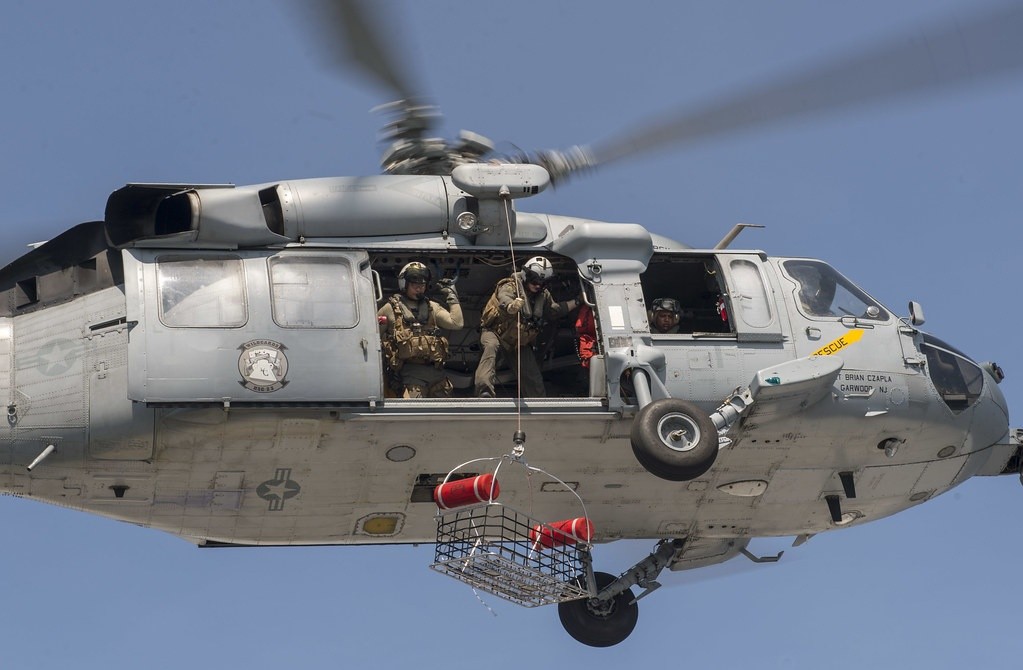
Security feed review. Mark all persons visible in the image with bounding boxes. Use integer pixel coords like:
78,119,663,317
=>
378,262,464,398
574,304,599,397
798,278,836,317
648,297,681,333
475,256,591,398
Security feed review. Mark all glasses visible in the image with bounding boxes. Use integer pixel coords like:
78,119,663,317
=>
527,278,547,286
408,280,427,289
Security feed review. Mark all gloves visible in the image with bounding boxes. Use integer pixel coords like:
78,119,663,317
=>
575,288,592,306
436,278,460,304
510,298,525,311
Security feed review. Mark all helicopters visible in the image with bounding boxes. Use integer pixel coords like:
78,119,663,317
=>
0,0,1023,647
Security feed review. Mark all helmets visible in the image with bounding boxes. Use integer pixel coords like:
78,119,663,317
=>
398,262,432,291
521,256,554,287
648,298,683,326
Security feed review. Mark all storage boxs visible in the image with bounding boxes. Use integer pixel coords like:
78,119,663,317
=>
426,502,598,610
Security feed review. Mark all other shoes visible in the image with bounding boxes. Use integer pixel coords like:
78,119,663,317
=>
479,386,495,398
